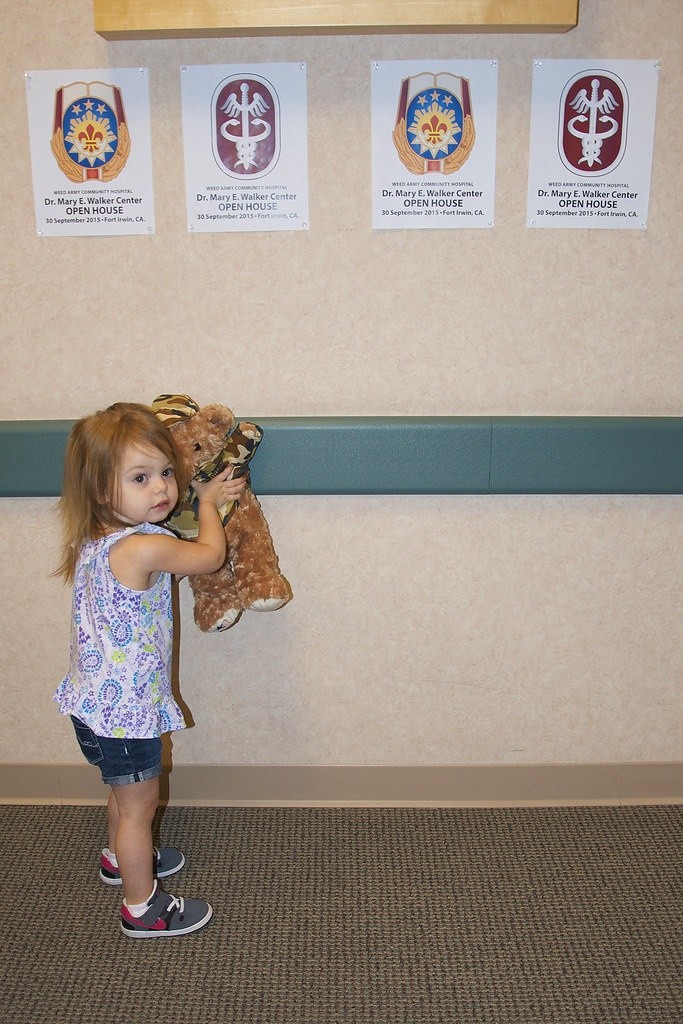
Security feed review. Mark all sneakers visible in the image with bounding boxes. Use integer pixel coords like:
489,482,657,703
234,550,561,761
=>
120,878,213,938
99,845,186,885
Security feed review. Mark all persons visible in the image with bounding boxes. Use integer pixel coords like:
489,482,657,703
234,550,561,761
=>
50,402,250,940
